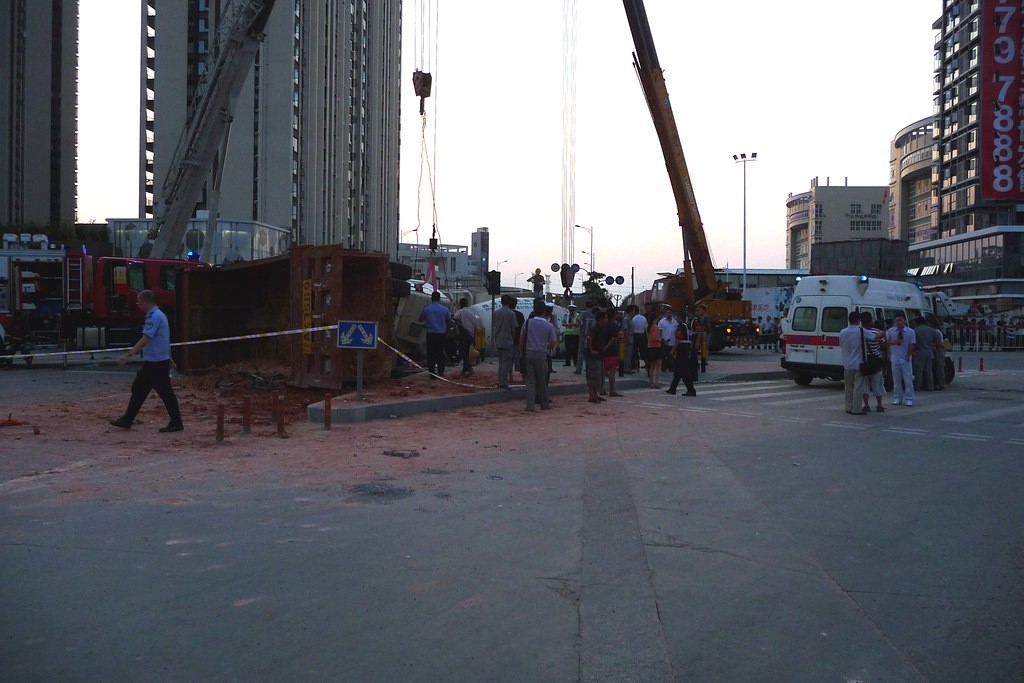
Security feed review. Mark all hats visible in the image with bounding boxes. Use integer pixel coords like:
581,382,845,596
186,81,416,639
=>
684,305,697,311
568,304,577,309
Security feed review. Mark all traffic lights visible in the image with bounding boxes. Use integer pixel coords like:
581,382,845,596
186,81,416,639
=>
483,270,500,296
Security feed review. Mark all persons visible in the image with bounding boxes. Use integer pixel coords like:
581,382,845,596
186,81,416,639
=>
561,305,580,367
943,313,1024,352
109,290,184,433
575,297,711,403
838,311,949,415
492,295,557,411
752,307,789,353
452,298,476,374
527,268,545,298
419,292,452,380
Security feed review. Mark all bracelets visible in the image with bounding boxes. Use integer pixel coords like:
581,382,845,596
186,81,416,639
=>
128,352,132,357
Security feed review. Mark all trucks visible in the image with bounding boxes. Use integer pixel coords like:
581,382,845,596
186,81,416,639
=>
175,243,411,387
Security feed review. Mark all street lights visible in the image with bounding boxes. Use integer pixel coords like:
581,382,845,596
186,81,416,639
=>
497,260,507,271
583,251,594,273
515,273,523,288
733,153,757,300
575,225,592,280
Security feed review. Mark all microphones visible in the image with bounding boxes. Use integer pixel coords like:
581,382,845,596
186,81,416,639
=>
897,332,903,345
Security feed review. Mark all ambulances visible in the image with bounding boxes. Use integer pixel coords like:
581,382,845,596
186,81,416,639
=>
781,275,943,386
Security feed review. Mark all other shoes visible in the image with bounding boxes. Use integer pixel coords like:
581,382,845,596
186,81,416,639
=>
893,398,902,404
160,420,183,432
430,368,557,411
863,406,870,411
109,419,131,429
563,362,624,403
878,405,884,411
905,400,912,405
650,364,707,396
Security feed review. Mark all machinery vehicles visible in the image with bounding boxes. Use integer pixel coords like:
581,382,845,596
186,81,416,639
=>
394,279,568,364
0,0,437,363
622,0,760,351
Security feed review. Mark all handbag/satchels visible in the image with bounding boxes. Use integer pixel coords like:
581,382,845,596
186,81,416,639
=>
860,362,872,374
469,342,480,360
520,356,526,373
